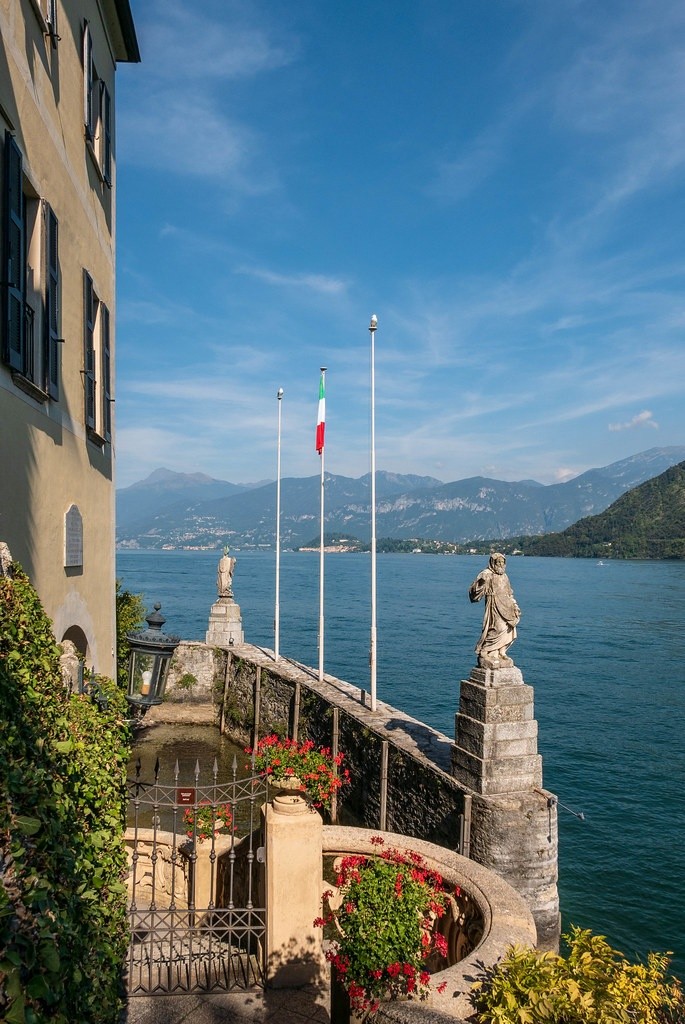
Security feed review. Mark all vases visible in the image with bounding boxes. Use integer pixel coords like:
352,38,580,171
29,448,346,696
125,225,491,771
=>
268,772,304,789
198,818,224,830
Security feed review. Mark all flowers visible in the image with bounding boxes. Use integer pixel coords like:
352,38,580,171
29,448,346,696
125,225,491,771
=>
182,800,238,844
312,837,461,1019
245,734,351,813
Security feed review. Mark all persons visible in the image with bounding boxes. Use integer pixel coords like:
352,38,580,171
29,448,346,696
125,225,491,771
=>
469,553,521,659
216,546,236,604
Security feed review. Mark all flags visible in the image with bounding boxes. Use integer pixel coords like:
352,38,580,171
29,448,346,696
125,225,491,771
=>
316,374,325,455
